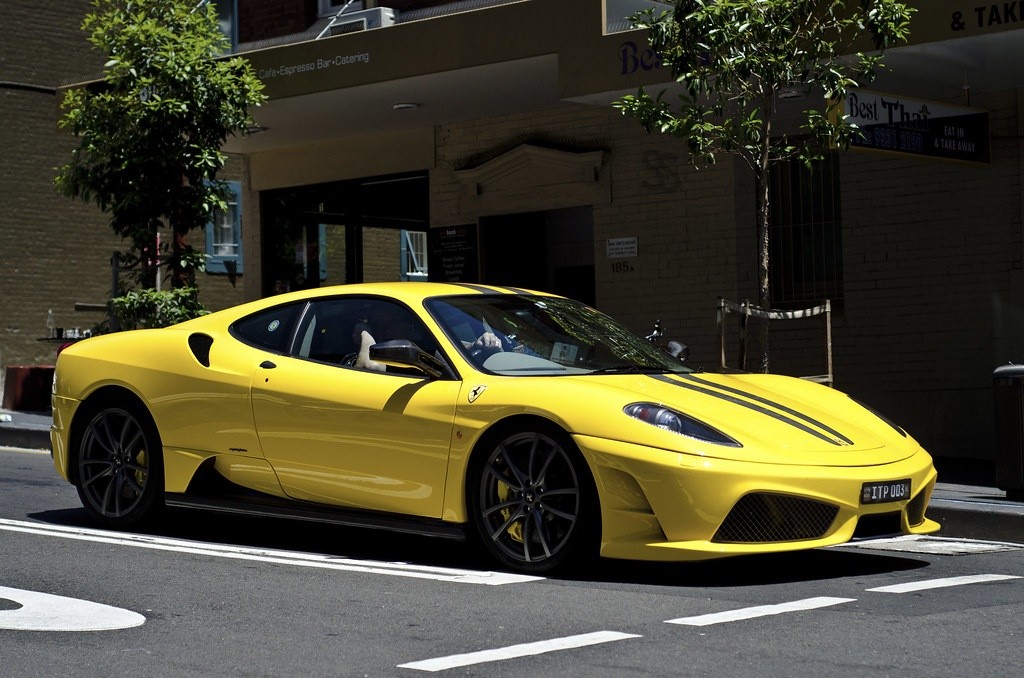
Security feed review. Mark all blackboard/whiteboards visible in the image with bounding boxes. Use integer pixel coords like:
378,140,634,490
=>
426,223,479,285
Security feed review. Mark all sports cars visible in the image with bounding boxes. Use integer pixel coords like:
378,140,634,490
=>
46,283,941,574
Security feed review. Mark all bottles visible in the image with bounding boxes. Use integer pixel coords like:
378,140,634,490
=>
45,310,54,338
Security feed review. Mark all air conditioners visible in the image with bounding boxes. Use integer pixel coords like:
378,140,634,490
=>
326,7,395,37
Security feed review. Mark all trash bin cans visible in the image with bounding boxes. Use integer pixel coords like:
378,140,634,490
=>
991,363,1024,503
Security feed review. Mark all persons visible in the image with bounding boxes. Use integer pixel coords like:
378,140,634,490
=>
352,306,503,374
